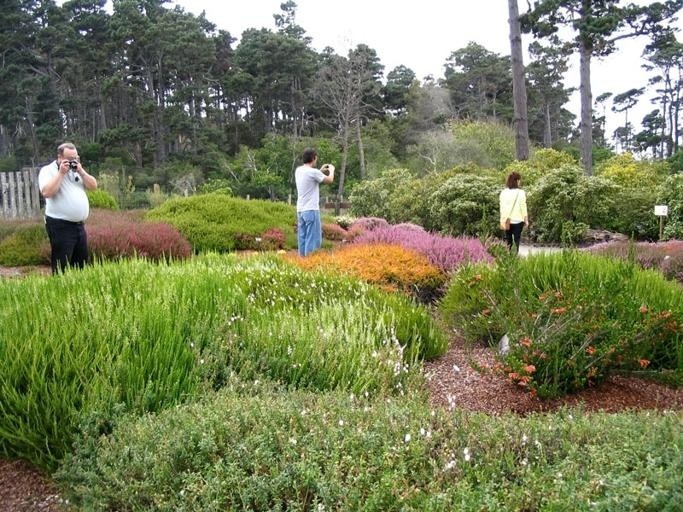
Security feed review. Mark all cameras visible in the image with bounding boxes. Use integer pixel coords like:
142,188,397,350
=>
65,161,78,169
325,166,329,168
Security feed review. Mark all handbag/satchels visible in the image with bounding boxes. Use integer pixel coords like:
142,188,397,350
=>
499,217,511,230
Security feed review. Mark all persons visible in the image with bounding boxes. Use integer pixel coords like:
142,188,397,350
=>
295,149,335,256
38,143,97,276
499,172,528,252
